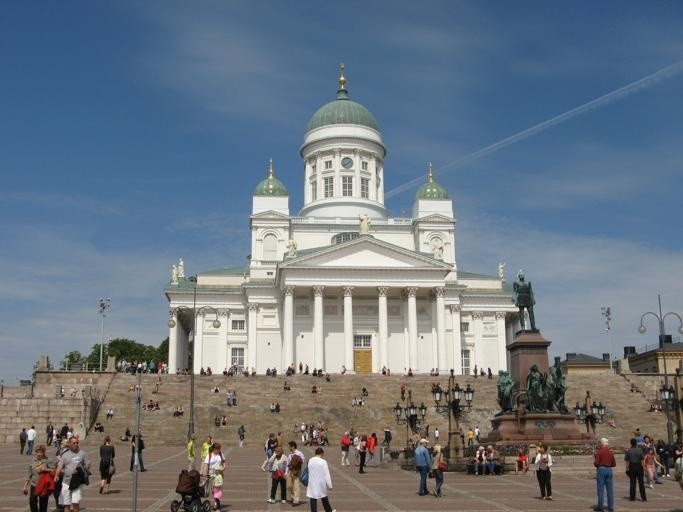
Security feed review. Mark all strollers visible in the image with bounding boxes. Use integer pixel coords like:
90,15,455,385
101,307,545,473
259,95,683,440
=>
171,468,212,512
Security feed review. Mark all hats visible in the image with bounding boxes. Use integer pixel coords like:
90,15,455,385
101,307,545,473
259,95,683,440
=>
420,438,429,443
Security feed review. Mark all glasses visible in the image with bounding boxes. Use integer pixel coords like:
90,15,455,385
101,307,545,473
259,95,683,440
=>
72,442,79,445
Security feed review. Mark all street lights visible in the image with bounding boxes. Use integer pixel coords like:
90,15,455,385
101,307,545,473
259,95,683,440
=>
164,286,220,443
600,304,616,375
573,393,606,448
98,295,110,371
390,388,426,468
434,381,475,466
648,385,676,462
130,367,142,511
633,291,683,450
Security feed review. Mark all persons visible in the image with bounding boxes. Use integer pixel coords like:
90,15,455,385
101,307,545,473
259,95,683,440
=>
473,364,492,379
186,435,226,512
260,420,337,512
352,396,365,418
609,414,617,428
172,265,177,283
496,365,567,414
380,365,441,403
270,401,281,420
61,386,79,397
432,238,444,261
238,424,246,449
499,262,506,279
340,427,392,474
592,428,683,512
105,377,187,419
177,258,184,277
117,358,191,377
458,425,554,501
513,270,539,331
359,214,371,234
124,426,147,472
411,425,448,497
286,236,297,256
212,384,238,427
264,362,368,395
20,421,115,511
199,365,257,373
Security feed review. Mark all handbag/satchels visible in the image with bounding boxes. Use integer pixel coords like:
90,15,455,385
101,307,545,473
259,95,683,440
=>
108,464,115,475
300,469,308,484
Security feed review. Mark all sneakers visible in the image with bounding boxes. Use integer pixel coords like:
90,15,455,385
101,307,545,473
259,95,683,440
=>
265,498,275,504
326,509,337,512
290,503,300,507
547,495,552,501
592,506,604,512
431,489,439,499
280,499,286,504
648,484,655,489
539,497,545,501
606,508,614,512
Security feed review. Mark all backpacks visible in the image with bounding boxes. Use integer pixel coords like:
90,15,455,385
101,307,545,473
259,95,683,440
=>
437,463,446,472
